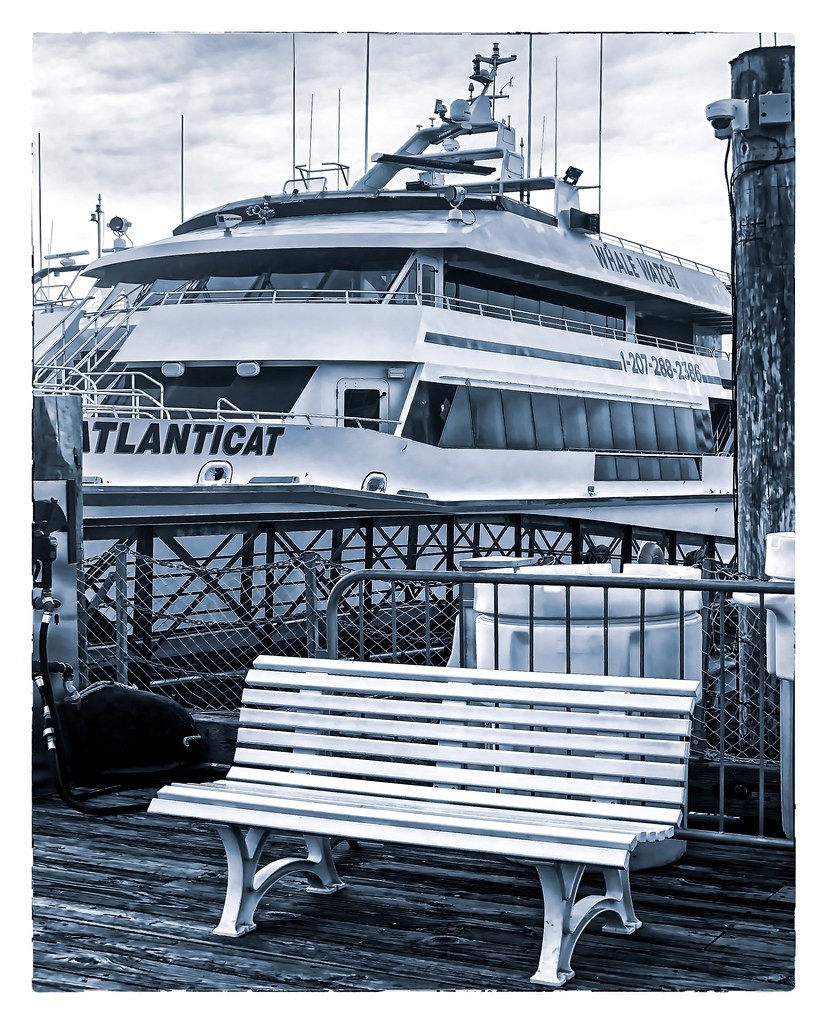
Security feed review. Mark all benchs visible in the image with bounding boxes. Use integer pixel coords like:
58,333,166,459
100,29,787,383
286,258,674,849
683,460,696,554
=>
147,651,702,986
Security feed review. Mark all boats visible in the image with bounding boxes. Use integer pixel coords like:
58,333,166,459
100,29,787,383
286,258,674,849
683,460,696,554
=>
33,29,739,652
